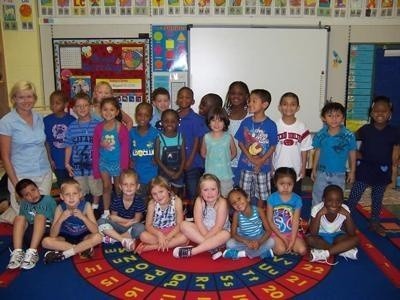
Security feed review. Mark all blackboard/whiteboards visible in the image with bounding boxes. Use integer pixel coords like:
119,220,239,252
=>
186,25,330,134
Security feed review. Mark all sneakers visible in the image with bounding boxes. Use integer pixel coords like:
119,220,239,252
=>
223,249,238,259
309,249,339,265
19,248,39,270
260,249,274,259
44,251,64,263
173,246,194,258
79,247,94,259
102,235,111,244
368,224,387,237
122,238,136,251
339,247,358,260
211,248,222,260
6,249,25,269
